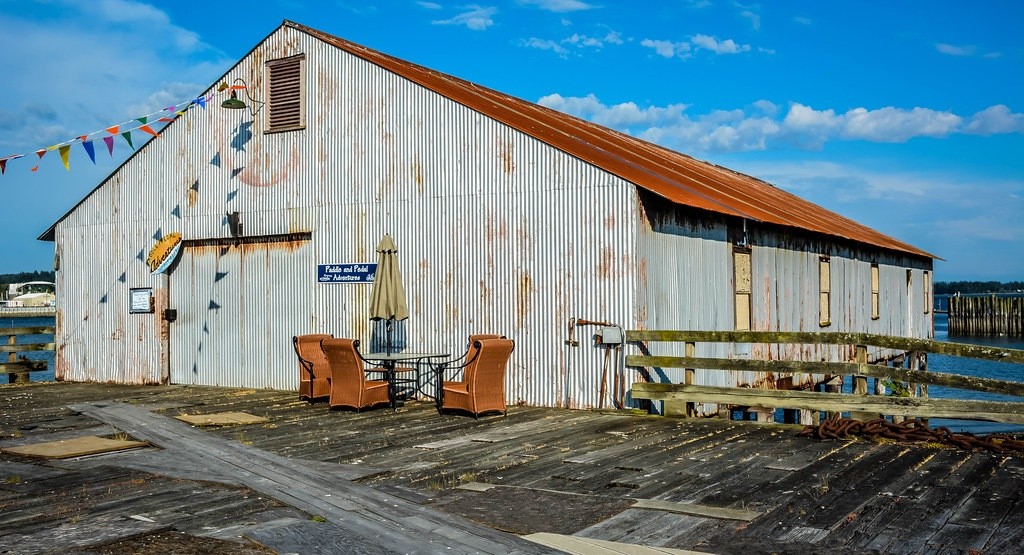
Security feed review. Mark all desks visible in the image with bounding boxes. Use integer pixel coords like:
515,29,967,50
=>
363,353,450,410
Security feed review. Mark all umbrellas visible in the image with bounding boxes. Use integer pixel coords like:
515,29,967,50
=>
370,233,409,355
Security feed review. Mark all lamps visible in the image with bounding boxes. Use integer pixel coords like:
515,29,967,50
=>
221,78,265,109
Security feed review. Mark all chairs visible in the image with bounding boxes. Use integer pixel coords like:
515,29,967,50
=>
293,334,391,413
435,334,515,421
